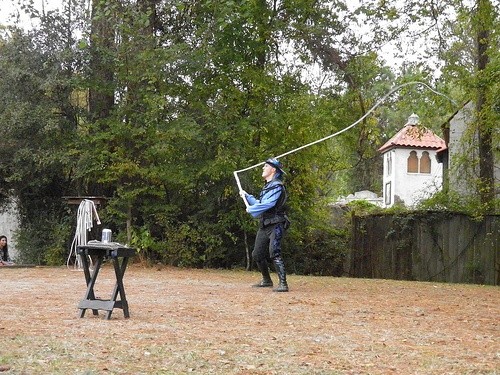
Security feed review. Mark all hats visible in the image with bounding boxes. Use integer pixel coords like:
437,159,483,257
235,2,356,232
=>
267,159,287,175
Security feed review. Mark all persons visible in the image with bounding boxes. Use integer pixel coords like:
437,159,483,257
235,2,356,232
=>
0,235,11,267
241,159,289,293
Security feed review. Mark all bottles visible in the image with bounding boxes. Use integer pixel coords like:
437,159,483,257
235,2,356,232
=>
101,228,111,244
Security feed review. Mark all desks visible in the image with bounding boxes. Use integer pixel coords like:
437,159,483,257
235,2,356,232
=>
75,247,136,317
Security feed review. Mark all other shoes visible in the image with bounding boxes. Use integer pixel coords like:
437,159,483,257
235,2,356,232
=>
254,280,273,287
272,285,288,292
